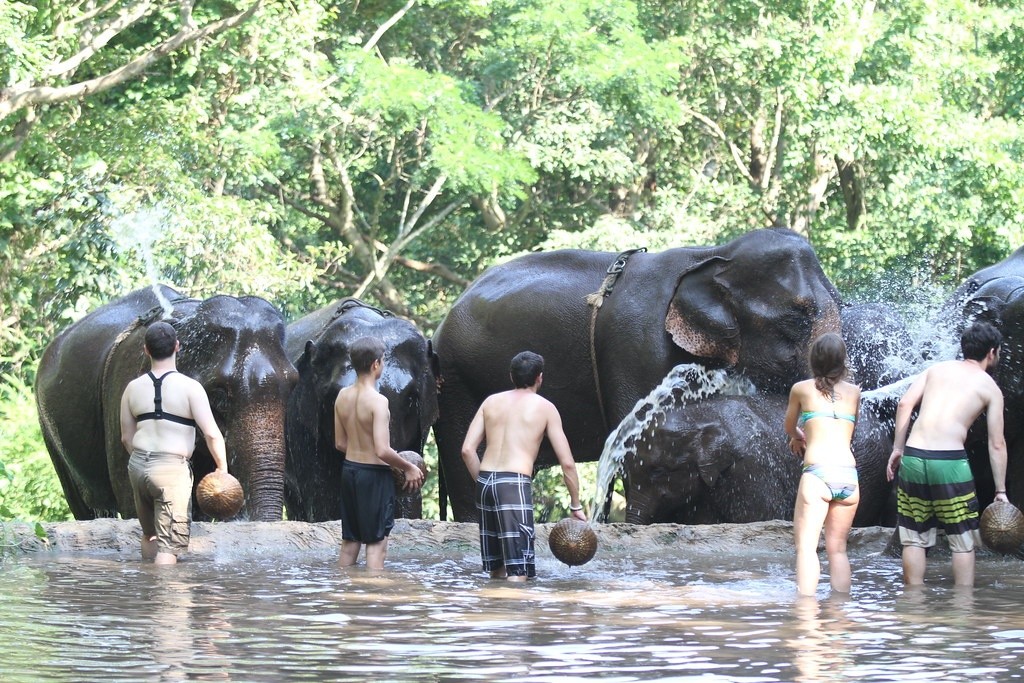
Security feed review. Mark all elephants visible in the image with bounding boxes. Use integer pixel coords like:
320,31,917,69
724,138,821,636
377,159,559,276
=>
598,244,1024,527
285,296,443,523
34,283,301,523
431,219,856,525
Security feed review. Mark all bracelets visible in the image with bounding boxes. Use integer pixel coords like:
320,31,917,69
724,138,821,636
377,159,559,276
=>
995,489,1006,494
796,434,806,442
893,449,905,453
568,504,583,511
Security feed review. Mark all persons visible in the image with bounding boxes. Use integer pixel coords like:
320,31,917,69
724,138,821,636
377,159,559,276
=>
887,322,1010,587
332,336,425,572
783,332,862,596
460,350,588,582
119,320,228,565
